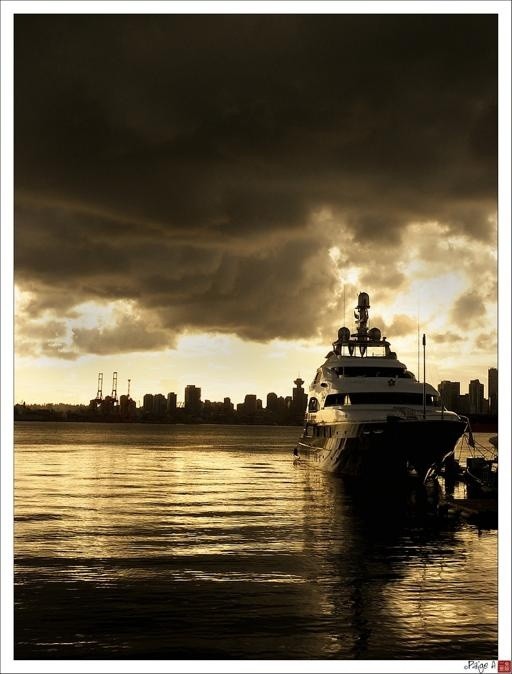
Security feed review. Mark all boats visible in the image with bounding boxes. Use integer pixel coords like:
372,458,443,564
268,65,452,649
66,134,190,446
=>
293,291,470,475
